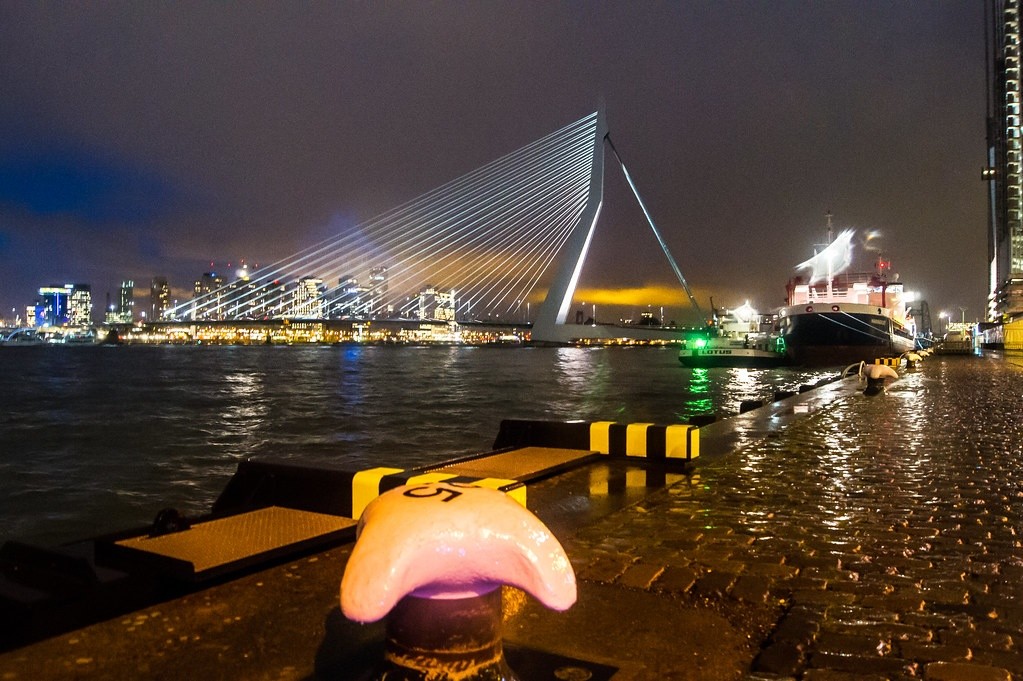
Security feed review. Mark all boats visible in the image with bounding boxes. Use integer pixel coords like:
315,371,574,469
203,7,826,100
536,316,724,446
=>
0,316,124,350
678,249,779,367
775,252,986,375
